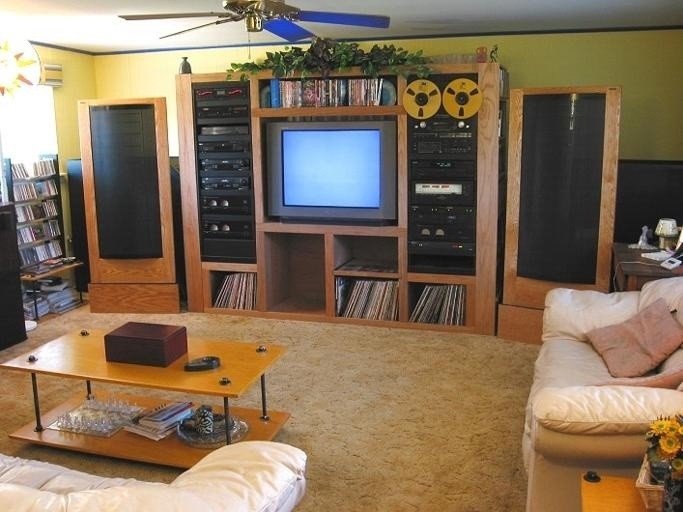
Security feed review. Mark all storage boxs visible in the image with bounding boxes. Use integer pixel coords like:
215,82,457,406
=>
103,320,188,369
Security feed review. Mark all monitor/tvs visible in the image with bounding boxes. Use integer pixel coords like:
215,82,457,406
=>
266,121,396,225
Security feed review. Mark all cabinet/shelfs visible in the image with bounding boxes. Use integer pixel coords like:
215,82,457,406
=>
173,63,499,336
2,152,66,267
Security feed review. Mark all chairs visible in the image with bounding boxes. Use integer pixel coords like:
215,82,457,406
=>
0,440,305,511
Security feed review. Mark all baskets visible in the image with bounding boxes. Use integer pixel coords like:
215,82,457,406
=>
635,450,667,511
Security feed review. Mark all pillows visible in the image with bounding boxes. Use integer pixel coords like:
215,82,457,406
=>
582,296,682,388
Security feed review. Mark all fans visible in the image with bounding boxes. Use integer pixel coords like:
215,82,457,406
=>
118,0,390,44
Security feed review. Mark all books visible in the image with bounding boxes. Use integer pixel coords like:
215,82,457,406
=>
124,399,195,443
271,78,384,108
212,273,256,311
335,276,465,325
10,158,84,319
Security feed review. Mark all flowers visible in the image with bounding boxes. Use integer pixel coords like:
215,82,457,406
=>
646,412,683,482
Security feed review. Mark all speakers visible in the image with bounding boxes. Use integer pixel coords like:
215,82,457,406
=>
77,97,177,284
502,84,622,311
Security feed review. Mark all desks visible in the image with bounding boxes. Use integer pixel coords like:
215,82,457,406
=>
20,260,86,322
611,242,682,291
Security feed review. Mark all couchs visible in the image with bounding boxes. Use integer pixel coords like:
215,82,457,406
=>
517,276,682,511
